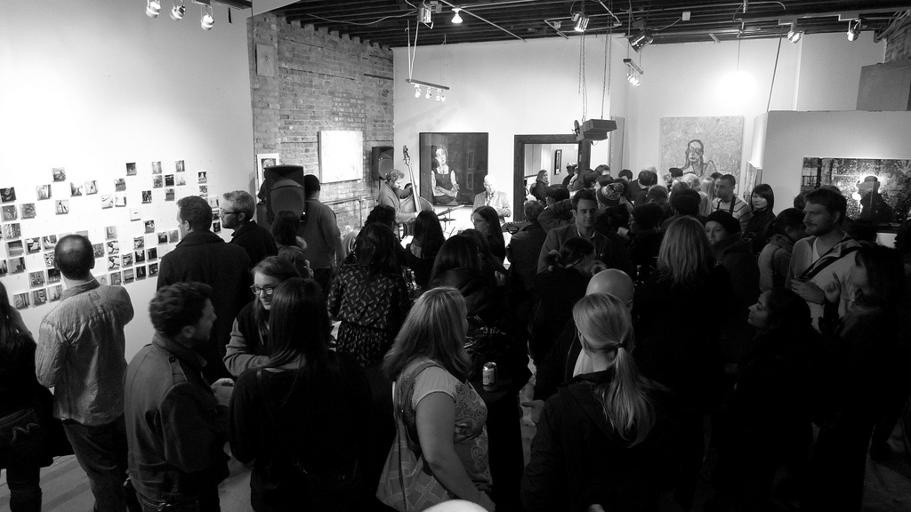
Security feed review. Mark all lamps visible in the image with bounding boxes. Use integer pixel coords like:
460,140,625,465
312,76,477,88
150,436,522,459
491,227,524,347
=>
451,6,464,25
629,31,655,54
787,23,807,46
572,10,591,35
407,79,451,103
838,11,866,43
623,58,645,88
144,0,216,32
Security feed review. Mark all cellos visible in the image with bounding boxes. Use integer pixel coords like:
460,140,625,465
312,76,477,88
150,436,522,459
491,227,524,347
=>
399,145,434,259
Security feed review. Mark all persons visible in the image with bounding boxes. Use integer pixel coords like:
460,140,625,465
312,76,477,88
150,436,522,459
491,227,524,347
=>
156,196,255,486
34,234,143,511
430,144,461,206
219,163,911,510
124,282,236,511
0,282,76,512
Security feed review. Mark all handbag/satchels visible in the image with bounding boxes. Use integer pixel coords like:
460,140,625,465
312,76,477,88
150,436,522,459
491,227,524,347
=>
375,418,455,512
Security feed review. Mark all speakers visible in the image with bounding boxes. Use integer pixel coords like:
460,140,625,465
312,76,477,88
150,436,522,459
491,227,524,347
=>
372,146,394,181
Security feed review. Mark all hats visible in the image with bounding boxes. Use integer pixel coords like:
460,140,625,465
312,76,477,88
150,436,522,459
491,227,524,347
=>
703,210,737,233
596,182,625,207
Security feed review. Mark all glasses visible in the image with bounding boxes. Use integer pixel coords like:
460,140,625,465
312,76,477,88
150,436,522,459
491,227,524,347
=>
217,208,242,214
250,282,281,296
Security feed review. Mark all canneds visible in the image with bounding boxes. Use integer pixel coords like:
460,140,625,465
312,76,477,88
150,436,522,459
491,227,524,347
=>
483,361,498,392
